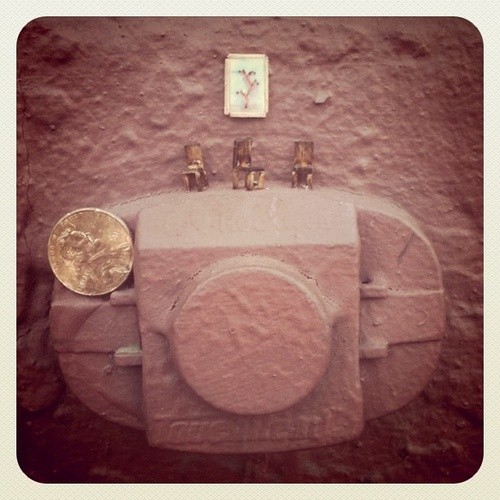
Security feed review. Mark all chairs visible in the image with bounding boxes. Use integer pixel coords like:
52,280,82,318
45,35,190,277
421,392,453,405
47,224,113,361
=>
180,142,207,191
231,138,264,190
291,141,313,189
